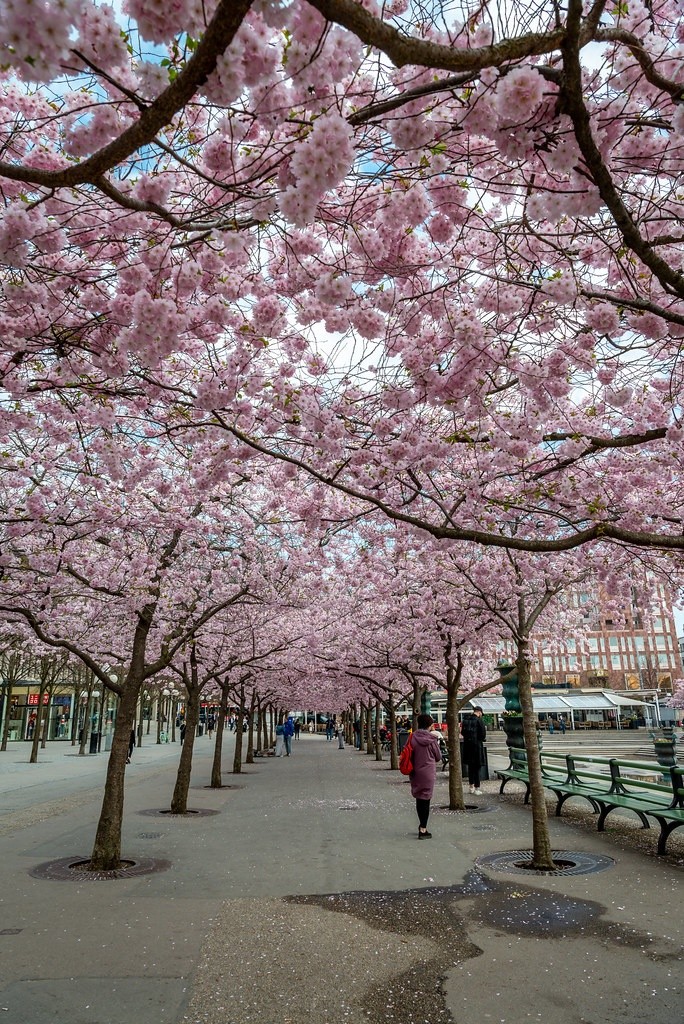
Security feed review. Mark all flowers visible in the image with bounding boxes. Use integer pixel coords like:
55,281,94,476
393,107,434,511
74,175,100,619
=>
653,738,672,744
503,710,518,717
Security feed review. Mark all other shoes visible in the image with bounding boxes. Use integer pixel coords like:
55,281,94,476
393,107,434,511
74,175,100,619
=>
419,830,432,840
469,786,482,796
342,731,345,735
126,758,131,764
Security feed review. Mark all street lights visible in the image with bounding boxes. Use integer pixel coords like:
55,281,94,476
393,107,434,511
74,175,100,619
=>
108,697,112,721
163,681,180,743
142,690,150,717
199,695,211,734
79,689,100,714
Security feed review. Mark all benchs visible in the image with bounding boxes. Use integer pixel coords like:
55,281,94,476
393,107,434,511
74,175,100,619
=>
519,777,564,804
548,785,608,814
647,808,684,856
590,794,668,830
540,751,601,814
567,753,650,829
612,759,683,807
508,746,564,800
494,770,528,794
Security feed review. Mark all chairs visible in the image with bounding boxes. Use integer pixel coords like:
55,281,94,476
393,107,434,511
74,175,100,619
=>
624,721,629,728
586,721,591,729
567,722,571,730
597,722,604,729
606,722,612,729
578,722,584,729
318,724,322,732
321,724,328,731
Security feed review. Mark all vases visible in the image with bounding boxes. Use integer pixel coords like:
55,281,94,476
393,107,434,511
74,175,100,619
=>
651,733,677,782
501,713,527,770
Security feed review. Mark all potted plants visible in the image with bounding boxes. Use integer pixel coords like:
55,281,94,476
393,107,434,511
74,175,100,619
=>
494,660,521,714
399,730,411,755
483,714,495,730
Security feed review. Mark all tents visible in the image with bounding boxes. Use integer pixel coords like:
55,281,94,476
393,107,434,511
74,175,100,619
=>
468,693,659,732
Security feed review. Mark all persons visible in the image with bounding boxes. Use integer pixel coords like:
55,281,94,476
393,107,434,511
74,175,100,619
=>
633,713,636,719
356,716,360,748
308,720,314,734
176,718,179,727
274,717,301,758
380,725,392,751
396,715,411,732
180,721,186,746
460,706,486,796
163,717,166,722
126,730,135,764
208,719,214,740
401,714,442,842
547,715,567,734
229,715,250,734
324,717,345,750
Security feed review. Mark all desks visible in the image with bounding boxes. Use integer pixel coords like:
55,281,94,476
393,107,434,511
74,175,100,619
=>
542,723,548,731
600,722,608,730
565,723,569,730
581,722,589,730
620,722,627,729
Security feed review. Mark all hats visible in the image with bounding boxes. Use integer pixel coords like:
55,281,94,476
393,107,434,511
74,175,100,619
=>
473,706,485,716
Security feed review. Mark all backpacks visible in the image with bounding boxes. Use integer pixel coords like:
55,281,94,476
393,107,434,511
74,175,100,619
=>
399,732,414,775
